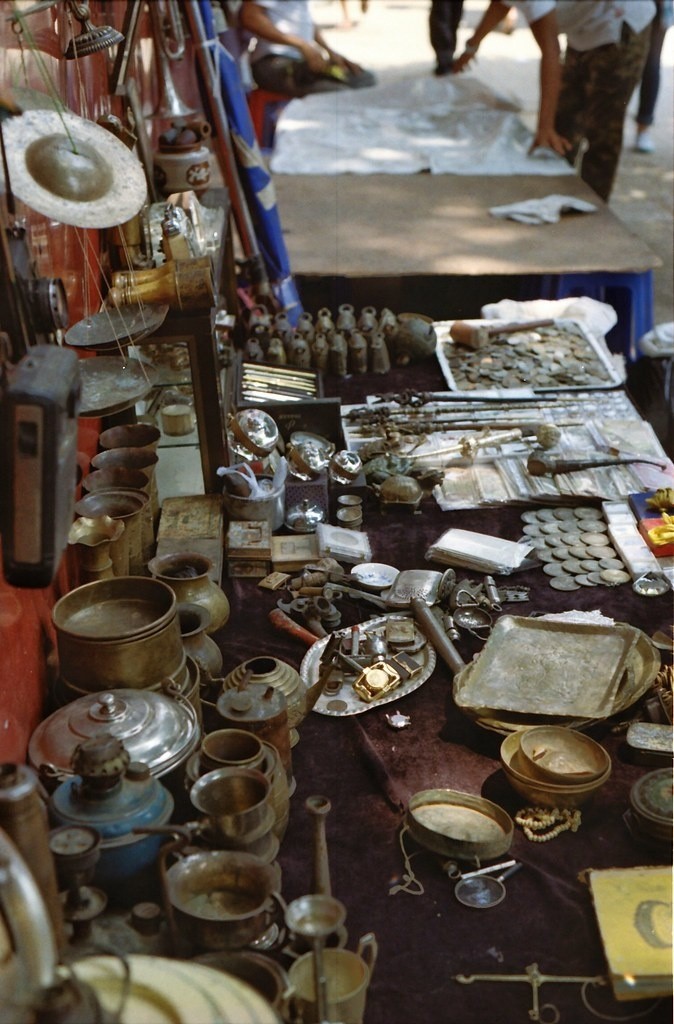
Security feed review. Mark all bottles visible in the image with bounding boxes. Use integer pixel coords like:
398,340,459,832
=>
154,142,210,199
164,204,202,258
162,219,190,263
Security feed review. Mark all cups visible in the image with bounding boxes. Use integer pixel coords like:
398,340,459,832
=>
289,934,377,1024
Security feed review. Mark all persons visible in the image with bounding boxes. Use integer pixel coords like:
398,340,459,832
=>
453,0,674,203
239,0,348,164
428,0,464,76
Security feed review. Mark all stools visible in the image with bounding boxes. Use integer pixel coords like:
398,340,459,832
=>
515,271,652,363
249,88,295,153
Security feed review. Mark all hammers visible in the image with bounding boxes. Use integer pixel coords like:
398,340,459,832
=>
449,317,554,348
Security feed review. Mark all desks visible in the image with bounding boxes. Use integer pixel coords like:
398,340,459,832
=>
60,315,672,1024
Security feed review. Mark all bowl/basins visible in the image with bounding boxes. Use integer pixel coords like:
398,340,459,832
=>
518,726,609,786
500,728,612,810
223,474,284,522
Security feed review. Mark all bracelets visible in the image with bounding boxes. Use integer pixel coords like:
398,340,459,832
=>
465,40,479,52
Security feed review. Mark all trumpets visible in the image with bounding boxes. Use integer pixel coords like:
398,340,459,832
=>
143,0,200,120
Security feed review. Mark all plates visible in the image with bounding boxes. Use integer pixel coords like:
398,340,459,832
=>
351,563,400,589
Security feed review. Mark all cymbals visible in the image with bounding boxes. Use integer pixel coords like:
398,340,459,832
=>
0,84,152,228
